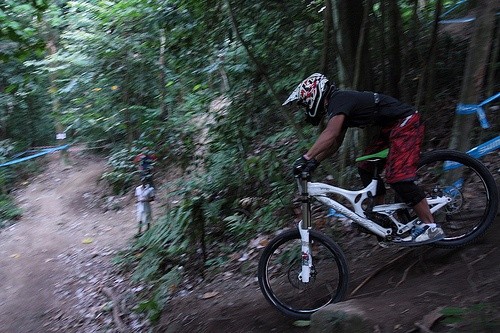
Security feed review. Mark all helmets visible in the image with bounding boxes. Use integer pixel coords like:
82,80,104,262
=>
282,73,337,126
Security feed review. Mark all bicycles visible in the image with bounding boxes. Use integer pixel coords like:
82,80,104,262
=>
258,147,499,320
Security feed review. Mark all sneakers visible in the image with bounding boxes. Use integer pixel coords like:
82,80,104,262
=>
400,221,446,246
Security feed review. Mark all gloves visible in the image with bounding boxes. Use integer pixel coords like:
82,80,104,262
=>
304,158,320,173
293,155,310,175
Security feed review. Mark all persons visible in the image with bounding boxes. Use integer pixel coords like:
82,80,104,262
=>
58,137,72,170
133,147,156,201
134,177,154,240
283,72,447,246
